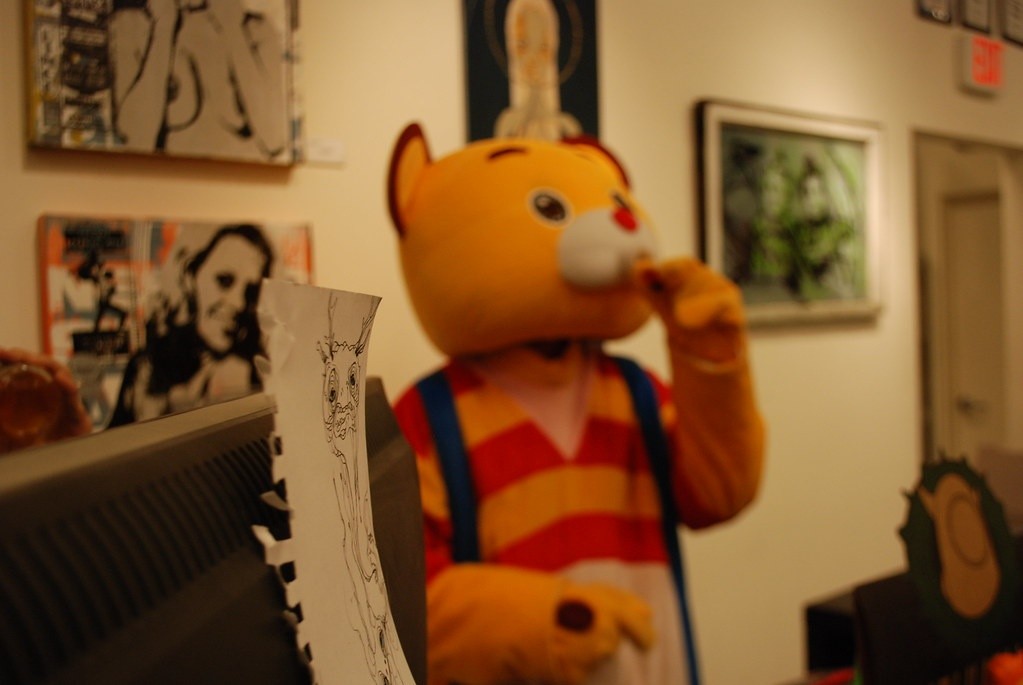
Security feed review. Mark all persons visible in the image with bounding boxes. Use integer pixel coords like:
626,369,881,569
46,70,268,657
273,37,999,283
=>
58,218,278,439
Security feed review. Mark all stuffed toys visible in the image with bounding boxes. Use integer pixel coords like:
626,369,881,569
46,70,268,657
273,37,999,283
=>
380,121,763,685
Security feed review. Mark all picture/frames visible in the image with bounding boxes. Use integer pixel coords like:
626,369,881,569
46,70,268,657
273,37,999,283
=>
959,0,993,35
18,0,303,173
997,0,1023,48
915,0,953,26
35,212,317,434
691,97,888,331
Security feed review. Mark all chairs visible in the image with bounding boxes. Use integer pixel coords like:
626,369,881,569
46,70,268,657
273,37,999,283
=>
858,534,1023,685
